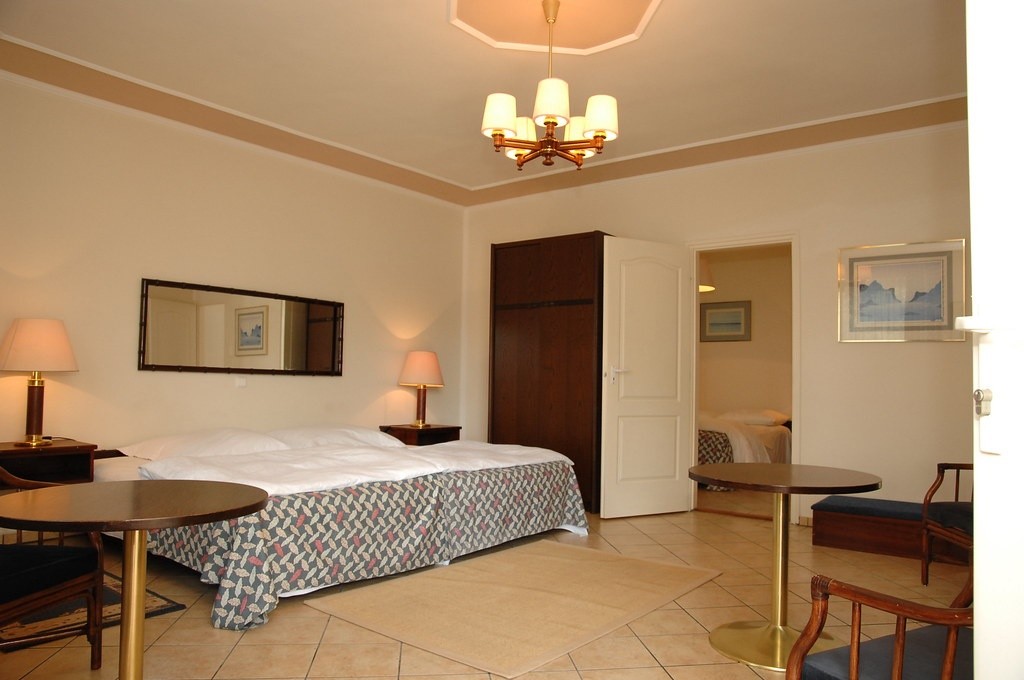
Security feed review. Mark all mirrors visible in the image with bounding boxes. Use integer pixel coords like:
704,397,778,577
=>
141,277,344,378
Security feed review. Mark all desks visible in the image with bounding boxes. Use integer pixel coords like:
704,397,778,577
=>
0,480,270,679
690,465,881,673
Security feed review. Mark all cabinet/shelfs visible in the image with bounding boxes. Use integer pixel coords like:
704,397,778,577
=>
489,229,616,513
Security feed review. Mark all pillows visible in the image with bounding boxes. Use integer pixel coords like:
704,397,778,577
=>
268,424,407,448
716,409,789,427
116,428,288,461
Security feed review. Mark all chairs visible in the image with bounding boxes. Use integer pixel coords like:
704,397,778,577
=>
786,570,973,680
1,470,103,671
920,464,973,586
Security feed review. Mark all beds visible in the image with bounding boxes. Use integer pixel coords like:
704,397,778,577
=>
697,424,793,491
90,442,589,631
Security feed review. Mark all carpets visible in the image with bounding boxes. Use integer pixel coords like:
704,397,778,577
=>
0,571,187,653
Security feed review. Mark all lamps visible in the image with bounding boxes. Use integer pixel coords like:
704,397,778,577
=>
396,350,445,429
0,316,79,448
481,1,619,172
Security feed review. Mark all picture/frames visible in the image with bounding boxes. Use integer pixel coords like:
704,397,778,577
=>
233,305,269,356
700,300,751,342
837,238,965,343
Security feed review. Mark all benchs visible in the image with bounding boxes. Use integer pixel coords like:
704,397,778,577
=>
811,496,923,559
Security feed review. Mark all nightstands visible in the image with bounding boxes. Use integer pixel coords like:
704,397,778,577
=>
379,424,462,447
0,440,98,482
